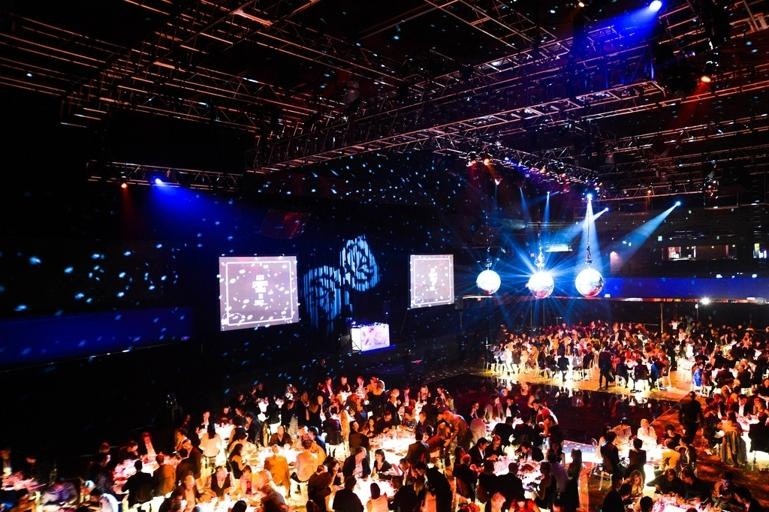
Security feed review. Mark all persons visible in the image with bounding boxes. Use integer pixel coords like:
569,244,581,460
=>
1,319,769,512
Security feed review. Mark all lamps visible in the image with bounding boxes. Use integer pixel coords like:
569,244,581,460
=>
473,196,603,296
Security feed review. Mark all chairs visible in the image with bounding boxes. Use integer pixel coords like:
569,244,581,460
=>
480,354,669,391
